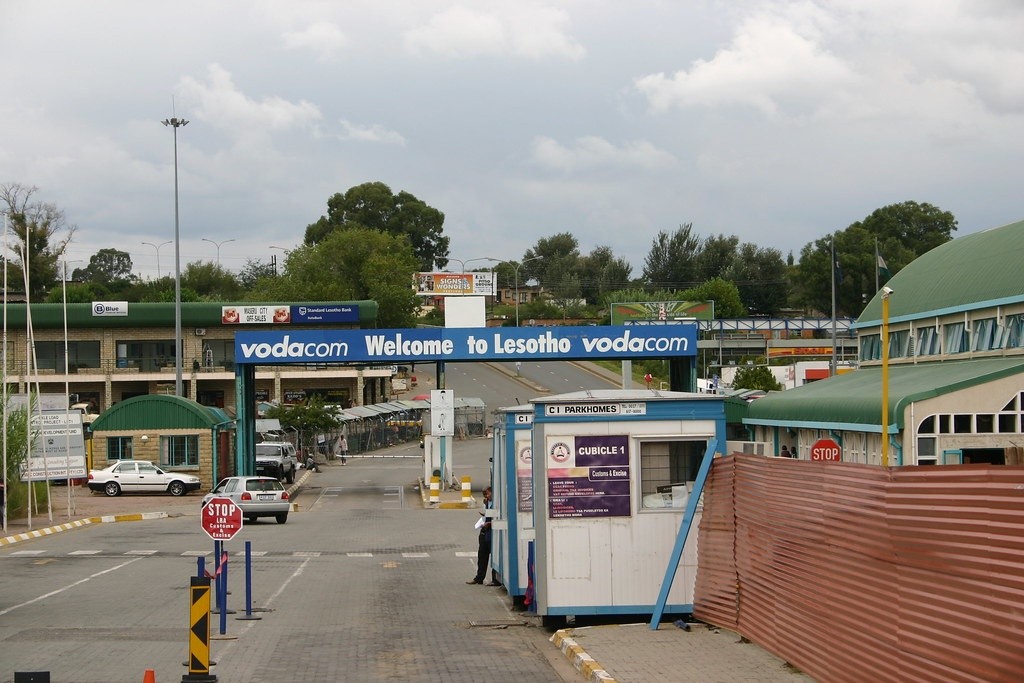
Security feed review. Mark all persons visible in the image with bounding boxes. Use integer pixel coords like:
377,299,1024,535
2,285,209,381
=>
306,454,323,473
438,391,448,431
780,445,797,458
465,485,493,585
644,371,652,390
339,434,348,466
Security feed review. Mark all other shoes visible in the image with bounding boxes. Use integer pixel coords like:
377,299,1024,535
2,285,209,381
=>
316,470,322,473
466,578,483,585
340,463,346,466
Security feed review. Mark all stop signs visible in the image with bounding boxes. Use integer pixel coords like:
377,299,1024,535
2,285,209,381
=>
201,497,243,541
810,438,842,462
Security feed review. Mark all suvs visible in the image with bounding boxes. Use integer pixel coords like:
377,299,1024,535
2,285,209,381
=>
256,441,298,484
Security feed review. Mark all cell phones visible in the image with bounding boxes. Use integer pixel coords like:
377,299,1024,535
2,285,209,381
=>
479,511,485,516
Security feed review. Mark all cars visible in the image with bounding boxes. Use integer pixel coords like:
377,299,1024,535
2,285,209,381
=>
69,403,100,433
88,460,201,498
202,475,291,525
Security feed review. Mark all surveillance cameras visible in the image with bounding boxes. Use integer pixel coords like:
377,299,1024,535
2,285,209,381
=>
882,287,893,294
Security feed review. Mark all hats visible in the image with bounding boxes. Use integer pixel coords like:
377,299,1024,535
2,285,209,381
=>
309,454,314,456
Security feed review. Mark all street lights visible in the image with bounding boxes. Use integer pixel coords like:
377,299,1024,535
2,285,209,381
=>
201,238,236,270
3,212,52,534
434,256,493,297
488,256,543,327
160,118,189,396
62,260,84,520
141,241,174,279
